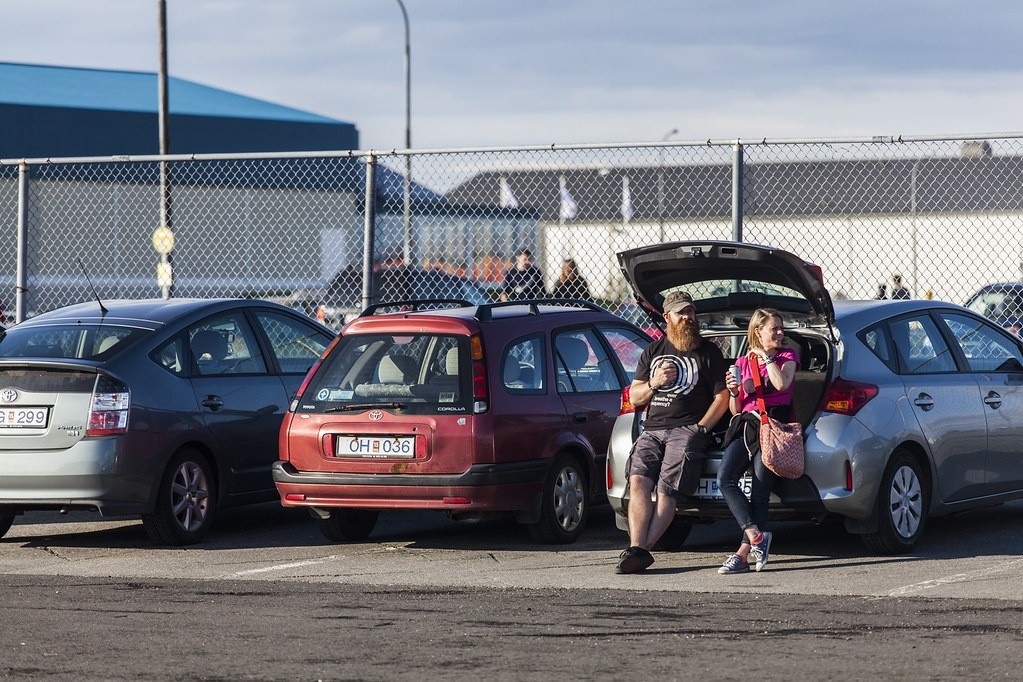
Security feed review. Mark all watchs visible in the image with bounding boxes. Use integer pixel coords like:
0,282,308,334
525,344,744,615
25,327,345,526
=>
697,424,708,435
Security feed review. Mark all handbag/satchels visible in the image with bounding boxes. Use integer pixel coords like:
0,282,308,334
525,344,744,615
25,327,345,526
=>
759,410,805,479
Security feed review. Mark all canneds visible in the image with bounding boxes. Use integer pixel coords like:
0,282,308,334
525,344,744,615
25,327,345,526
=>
661,361,672,367
729,364,740,386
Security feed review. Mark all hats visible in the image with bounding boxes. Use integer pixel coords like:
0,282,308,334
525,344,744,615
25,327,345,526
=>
664,291,697,314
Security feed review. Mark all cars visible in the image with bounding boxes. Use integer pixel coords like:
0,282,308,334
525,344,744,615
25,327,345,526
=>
317,266,487,331
923,282,1023,357
0,298,362,547
604,238,1023,554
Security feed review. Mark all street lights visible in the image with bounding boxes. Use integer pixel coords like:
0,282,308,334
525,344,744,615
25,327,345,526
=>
659,130,680,243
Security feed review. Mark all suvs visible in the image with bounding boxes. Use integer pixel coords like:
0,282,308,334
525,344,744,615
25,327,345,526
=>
271,297,657,544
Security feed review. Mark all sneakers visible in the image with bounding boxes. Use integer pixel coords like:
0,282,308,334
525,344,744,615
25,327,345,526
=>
613,547,655,574
751,531,772,572
717,553,750,573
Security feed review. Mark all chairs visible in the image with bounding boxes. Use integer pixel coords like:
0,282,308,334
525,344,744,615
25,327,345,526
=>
502,356,527,385
371,354,419,385
430,346,461,384
190,330,229,374
555,338,599,391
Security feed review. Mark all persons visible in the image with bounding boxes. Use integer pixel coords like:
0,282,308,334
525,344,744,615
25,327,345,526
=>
615,291,728,575
875,276,932,300
501,249,546,302
716,309,798,574
553,259,594,302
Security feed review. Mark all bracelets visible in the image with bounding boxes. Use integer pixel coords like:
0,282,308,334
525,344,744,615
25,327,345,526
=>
648,380,658,394
729,391,740,398
763,355,775,365
